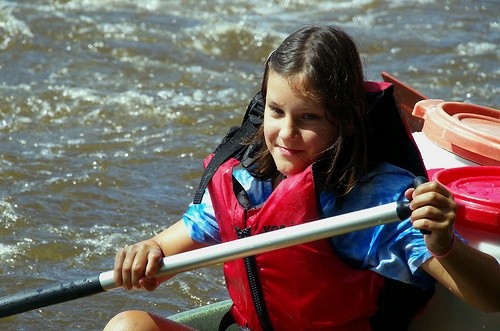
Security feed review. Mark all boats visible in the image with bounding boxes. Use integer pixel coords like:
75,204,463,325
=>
166,69,500,331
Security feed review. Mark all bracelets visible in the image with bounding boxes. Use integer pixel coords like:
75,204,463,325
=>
431,232,457,258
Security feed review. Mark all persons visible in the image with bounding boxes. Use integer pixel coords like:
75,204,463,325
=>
102,23,500,331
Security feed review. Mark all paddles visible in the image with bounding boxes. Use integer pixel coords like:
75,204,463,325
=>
0,175,431,317
